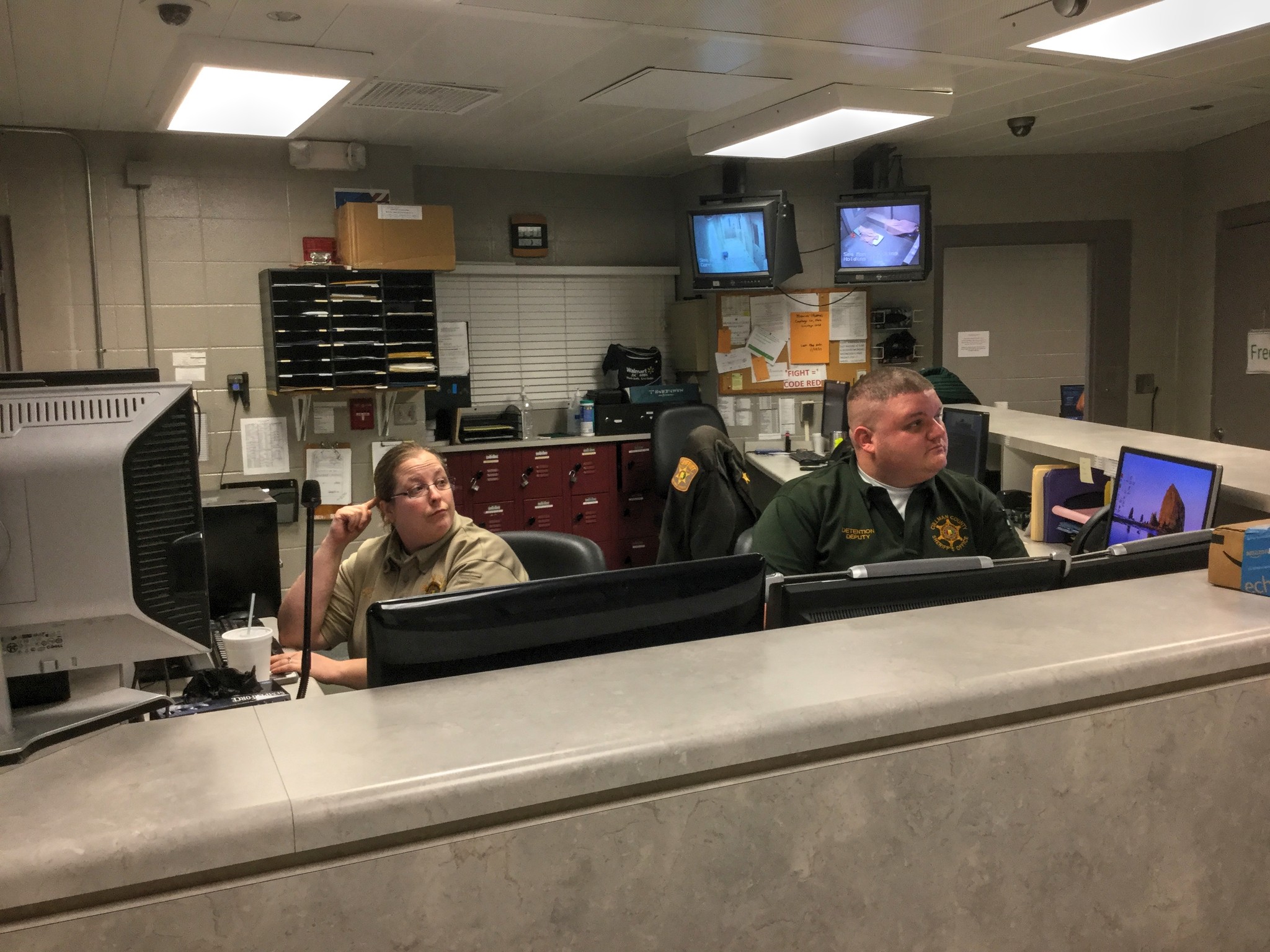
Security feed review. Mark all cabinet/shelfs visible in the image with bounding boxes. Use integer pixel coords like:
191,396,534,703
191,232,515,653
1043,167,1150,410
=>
257,269,441,396
431,428,661,573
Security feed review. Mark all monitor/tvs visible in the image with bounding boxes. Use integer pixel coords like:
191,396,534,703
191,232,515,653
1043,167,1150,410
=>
687,197,802,291
834,187,933,284
1105,446,1224,548
766,552,1072,630
365,553,767,696
1,383,211,678
0,369,160,390
938,407,989,484
1062,528,1215,590
820,379,849,440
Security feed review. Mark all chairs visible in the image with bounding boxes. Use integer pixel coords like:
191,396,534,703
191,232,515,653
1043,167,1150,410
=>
653,404,731,544
496,531,608,581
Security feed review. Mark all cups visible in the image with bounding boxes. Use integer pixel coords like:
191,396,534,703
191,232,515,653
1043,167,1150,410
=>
222,626,273,683
812,433,826,453
994,401,1008,410
830,430,846,454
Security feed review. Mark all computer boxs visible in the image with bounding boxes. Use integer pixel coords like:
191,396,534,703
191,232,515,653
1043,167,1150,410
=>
199,485,283,619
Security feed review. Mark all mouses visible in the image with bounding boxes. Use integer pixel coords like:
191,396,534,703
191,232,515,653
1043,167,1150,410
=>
220,610,255,619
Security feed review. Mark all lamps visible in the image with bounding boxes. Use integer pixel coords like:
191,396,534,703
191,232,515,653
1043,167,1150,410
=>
687,82,955,161
143,32,372,140
1000,0,1270,65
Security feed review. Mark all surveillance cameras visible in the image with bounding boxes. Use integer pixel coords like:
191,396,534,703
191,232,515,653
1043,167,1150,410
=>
157,4,194,27
1006,115,1036,138
1052,0,1090,20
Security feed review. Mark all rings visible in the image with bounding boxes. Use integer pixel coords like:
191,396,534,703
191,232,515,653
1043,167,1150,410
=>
287,656,291,664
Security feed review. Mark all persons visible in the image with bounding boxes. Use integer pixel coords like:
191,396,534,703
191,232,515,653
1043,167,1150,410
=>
750,365,1031,633
270,439,531,691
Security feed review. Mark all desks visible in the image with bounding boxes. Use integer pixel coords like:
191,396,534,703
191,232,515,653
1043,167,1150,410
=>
141,617,324,723
745,440,1072,577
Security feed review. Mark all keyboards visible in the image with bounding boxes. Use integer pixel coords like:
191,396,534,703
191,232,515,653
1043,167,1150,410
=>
789,450,826,467
207,613,299,688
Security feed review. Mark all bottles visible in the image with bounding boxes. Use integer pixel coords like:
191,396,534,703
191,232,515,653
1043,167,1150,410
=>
516,393,534,440
568,389,584,434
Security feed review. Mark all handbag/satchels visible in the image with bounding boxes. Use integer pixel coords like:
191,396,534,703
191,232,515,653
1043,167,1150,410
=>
602,343,663,390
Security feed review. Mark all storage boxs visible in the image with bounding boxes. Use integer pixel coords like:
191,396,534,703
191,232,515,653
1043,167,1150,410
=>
200,486,282,620
335,202,456,272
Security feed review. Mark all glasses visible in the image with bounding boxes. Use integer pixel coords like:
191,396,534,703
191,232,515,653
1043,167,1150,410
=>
387,475,457,502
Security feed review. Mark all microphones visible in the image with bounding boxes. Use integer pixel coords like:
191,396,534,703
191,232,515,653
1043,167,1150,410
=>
294,478,323,699
